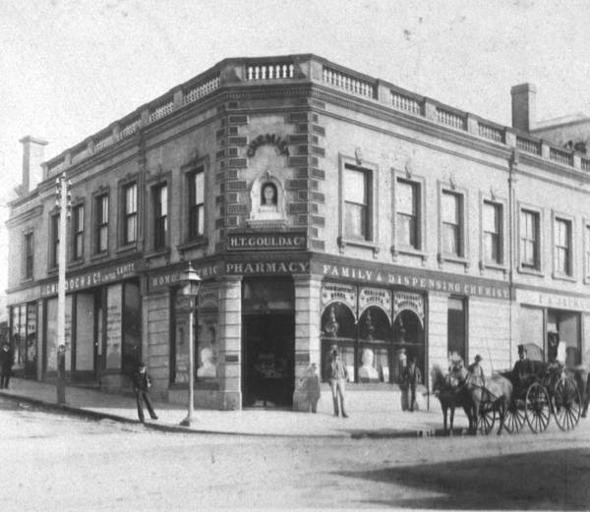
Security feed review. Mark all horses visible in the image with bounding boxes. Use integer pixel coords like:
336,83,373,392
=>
444,357,514,436
431,366,475,436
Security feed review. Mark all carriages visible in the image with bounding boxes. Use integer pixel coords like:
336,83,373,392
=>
431,342,580,436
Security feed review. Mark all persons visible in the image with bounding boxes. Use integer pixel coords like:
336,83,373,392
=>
195,347,217,379
467,354,486,377
357,348,382,379
403,355,423,411
1,341,14,391
326,348,349,418
132,362,160,423
507,342,537,407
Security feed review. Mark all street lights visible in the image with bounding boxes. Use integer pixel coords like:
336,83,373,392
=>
179,259,200,425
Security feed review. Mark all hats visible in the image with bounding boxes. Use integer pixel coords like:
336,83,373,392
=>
517,345,527,354
